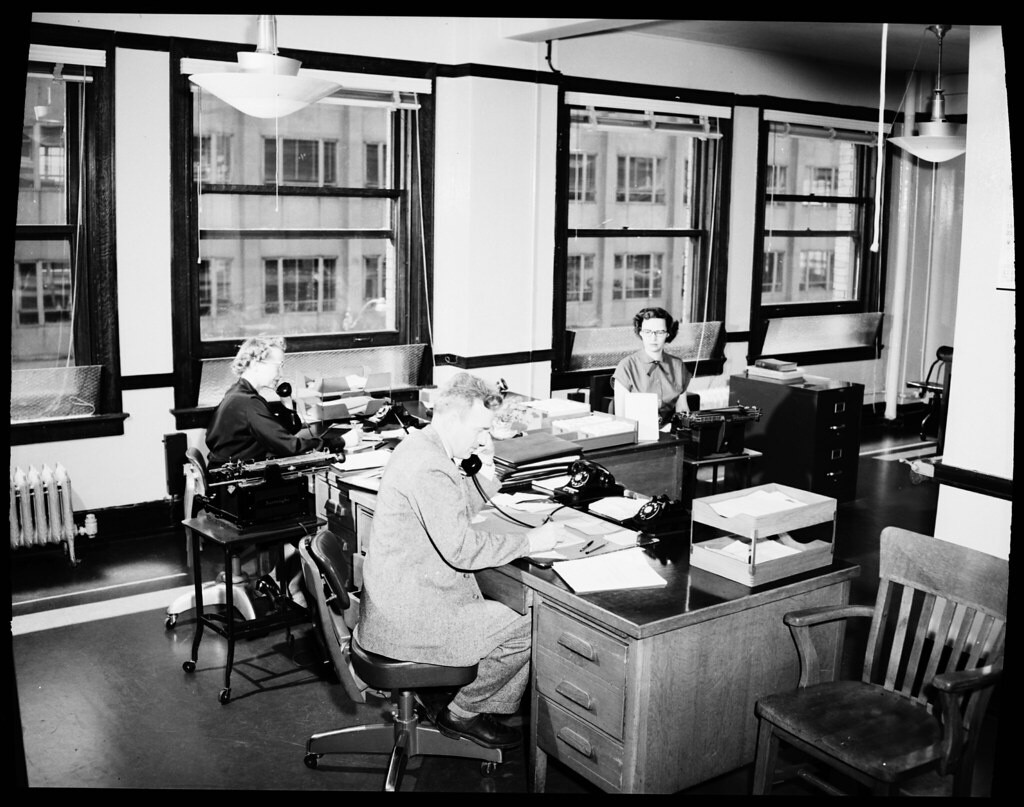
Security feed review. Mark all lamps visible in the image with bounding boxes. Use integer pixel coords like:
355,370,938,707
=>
886,25,966,163
188,0,342,119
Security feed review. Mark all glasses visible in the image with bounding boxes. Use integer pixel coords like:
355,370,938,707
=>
641,329,667,337
256,358,287,371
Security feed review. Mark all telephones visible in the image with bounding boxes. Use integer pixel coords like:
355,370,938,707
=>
367,400,421,429
630,493,692,538
553,459,625,509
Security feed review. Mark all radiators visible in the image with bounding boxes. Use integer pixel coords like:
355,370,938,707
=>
10,461,76,566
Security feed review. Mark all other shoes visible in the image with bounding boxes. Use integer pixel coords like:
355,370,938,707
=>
494,714,523,727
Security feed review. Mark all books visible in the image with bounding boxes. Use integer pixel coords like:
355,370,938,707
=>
492,432,583,487
756,359,798,372
554,547,667,596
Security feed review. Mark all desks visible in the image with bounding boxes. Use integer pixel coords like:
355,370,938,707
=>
179,516,328,703
309,393,860,794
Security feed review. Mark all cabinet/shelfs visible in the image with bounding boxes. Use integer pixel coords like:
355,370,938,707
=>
689,483,837,588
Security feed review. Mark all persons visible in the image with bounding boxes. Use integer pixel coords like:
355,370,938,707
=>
610,307,691,415
354,374,563,749
206,333,324,623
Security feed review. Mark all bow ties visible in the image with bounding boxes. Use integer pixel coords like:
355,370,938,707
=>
647,360,669,377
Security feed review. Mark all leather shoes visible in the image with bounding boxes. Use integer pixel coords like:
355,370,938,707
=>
437,703,524,749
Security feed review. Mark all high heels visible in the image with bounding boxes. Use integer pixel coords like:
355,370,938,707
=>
275,596,313,619
254,574,282,602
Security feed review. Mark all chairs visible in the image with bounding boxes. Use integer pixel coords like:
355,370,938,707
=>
299,531,501,792
163,447,268,630
751,525,1009,796
